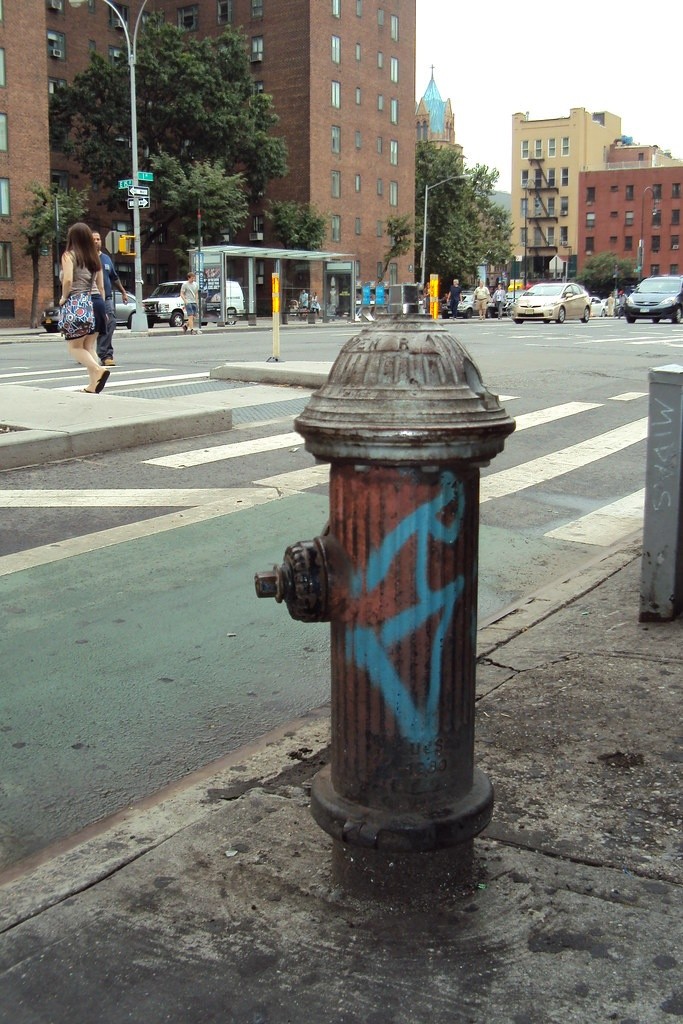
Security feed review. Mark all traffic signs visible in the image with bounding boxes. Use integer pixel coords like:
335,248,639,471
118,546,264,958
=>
127,198,150,209
128,185,150,197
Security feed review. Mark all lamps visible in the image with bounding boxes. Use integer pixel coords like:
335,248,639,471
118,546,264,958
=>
47,0,61,10
114,20,127,28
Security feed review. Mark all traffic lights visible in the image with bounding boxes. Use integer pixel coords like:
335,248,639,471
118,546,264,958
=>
118,238,131,254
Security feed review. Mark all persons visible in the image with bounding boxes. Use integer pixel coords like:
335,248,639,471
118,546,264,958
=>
446,279,463,319
423,282,430,313
618,291,627,320
91,230,128,365
308,292,321,314
59,223,110,394
180,272,198,331
607,294,614,317
492,284,506,319
473,281,491,320
299,289,309,309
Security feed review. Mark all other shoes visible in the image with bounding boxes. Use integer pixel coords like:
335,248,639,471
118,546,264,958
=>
479,317,482,320
100,359,115,366
182,325,187,334
191,331,197,335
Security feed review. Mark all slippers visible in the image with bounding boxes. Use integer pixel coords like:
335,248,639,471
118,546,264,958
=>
81,387,96,393
94,370,110,393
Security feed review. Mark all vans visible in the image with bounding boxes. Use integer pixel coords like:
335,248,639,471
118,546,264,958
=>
143,279,247,327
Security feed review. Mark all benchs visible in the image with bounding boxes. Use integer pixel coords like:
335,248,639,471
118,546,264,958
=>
282,311,317,324
227,312,259,325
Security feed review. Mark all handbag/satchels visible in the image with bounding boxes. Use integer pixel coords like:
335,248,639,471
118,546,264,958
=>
57,293,95,339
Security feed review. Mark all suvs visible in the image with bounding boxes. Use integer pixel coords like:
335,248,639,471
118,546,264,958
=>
624,274,683,324
39,289,146,332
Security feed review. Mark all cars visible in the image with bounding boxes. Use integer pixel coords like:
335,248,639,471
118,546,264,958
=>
440,282,610,324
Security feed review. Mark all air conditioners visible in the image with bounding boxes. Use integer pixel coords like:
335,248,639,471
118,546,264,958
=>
256,277,263,284
673,245,679,249
220,234,229,242
250,52,262,62
560,211,567,216
51,49,61,57
249,233,263,240
562,241,567,245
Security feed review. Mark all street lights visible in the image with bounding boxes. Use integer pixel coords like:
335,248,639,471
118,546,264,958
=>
420,174,468,277
638,187,657,282
523,179,540,287
69,0,150,332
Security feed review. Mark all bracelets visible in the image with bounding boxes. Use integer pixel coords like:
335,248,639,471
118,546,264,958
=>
62,296,66,301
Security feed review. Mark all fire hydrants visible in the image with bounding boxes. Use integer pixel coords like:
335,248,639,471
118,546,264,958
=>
253,320,517,854
